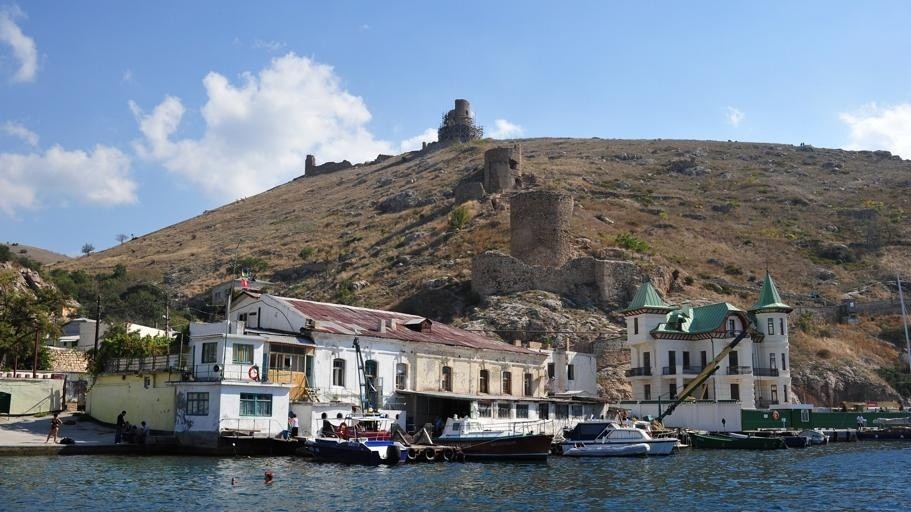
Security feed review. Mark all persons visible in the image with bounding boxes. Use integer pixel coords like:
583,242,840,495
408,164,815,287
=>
856,413,863,430
288,414,299,438
114,410,150,444
264,471,274,485
44,413,63,443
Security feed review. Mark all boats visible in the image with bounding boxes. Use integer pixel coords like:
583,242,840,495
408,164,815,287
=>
686,425,830,451
555,420,689,456
431,413,553,461
306,337,411,465
862,425,911,440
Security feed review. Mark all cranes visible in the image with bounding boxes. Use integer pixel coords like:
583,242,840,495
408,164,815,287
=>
652,321,765,436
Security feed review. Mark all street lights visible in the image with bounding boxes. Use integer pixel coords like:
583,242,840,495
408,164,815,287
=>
226,238,244,334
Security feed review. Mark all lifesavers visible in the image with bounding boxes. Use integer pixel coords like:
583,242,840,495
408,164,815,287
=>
249,366,259,380
425,447,435,460
408,448,416,460
338,423,347,437
444,448,454,460
